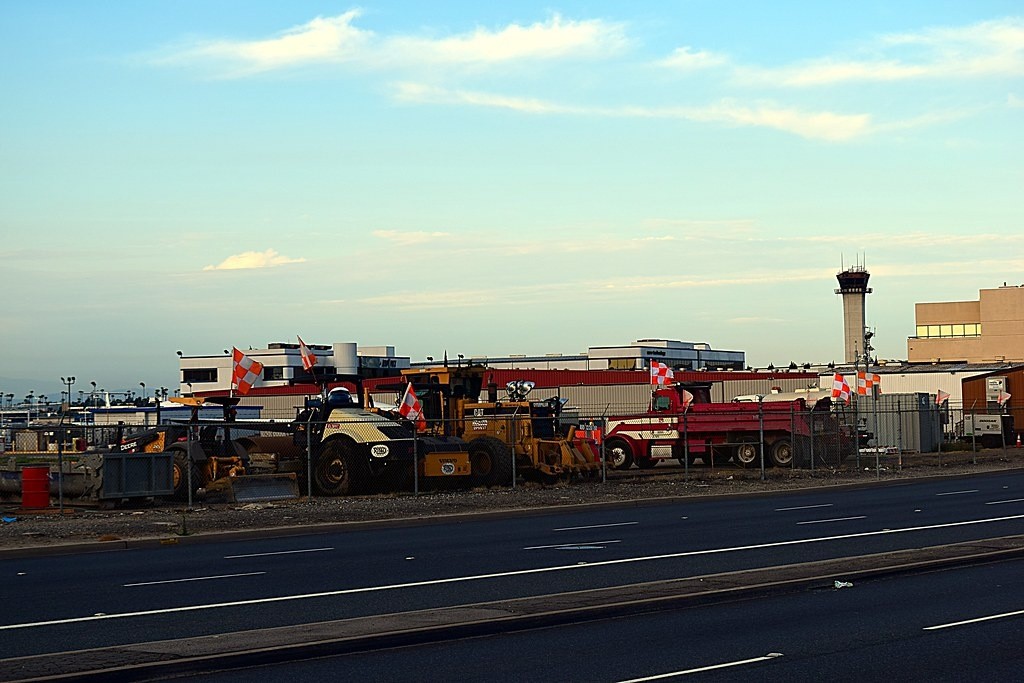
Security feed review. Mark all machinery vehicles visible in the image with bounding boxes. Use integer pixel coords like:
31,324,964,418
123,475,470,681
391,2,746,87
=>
106,355,876,509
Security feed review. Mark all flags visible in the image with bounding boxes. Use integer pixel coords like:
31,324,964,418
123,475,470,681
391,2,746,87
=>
399,384,427,431
997,390,1011,405
233,348,262,395
857,371,882,396
295,336,318,371
683,390,693,407
936,389,951,405
832,374,851,404
652,361,674,385
